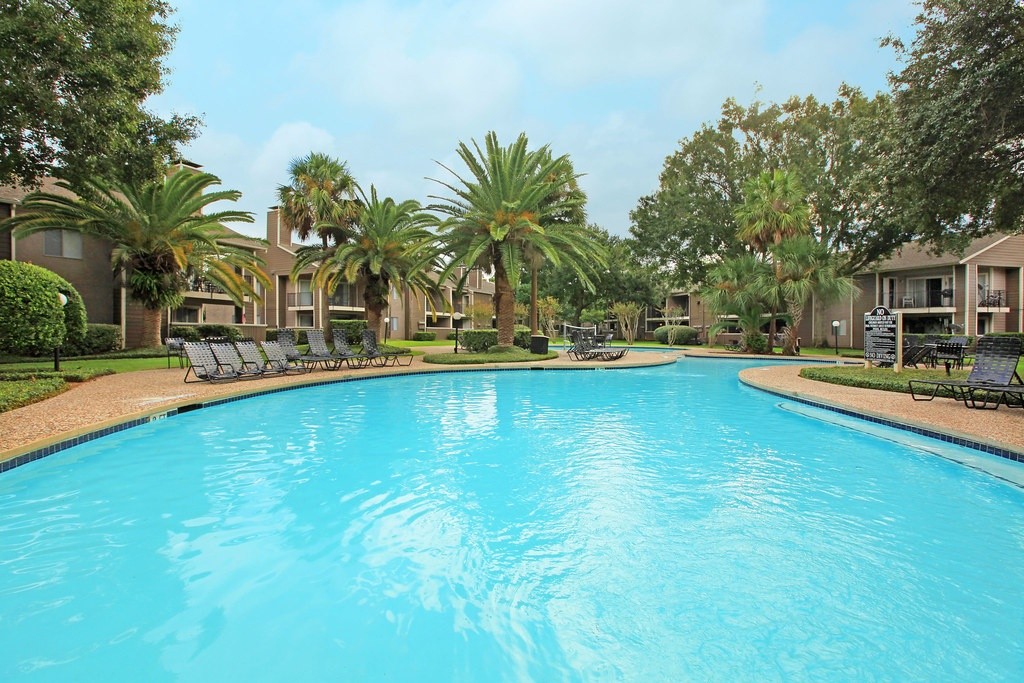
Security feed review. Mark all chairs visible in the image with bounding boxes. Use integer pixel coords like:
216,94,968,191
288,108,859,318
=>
164,328,413,385
908,337,1024,411
567,330,630,361
877,336,971,370
